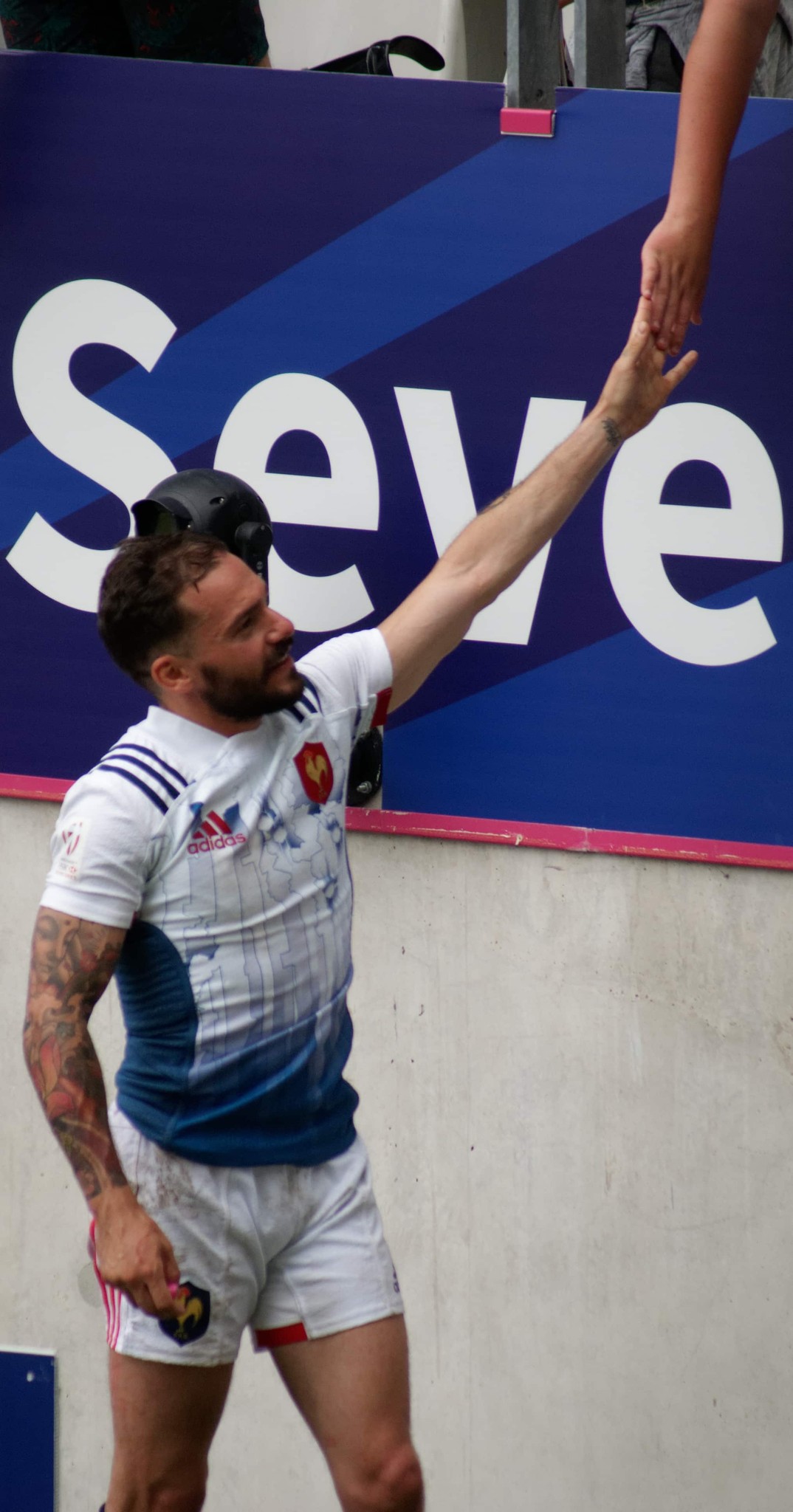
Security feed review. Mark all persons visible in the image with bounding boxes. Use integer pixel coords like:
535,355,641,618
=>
17,293,699,1511
641,0,781,358
